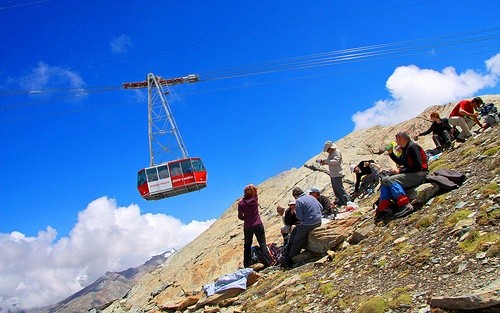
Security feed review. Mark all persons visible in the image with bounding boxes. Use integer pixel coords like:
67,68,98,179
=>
374,130,428,221
351,160,380,195
316,141,351,205
309,187,331,218
277,187,322,265
448,97,487,142
415,111,461,153
238,185,267,267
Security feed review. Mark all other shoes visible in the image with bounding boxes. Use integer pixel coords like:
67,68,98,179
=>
456,134,472,142
445,140,451,147
436,146,443,150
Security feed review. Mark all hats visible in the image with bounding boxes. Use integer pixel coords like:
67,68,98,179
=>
350,164,356,173
322,140,336,152
306,186,320,192
288,196,296,204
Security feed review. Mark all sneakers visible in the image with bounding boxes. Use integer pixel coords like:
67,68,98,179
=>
374,209,392,220
394,203,414,218
